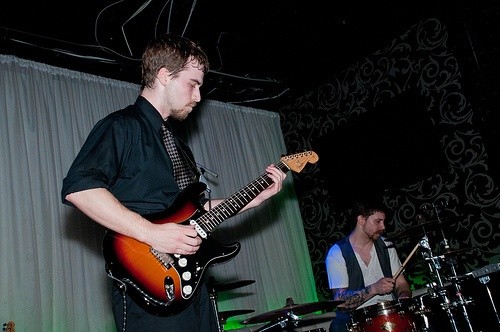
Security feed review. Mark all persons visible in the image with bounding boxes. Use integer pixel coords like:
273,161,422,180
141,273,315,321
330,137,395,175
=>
59,35,287,332
325,202,412,332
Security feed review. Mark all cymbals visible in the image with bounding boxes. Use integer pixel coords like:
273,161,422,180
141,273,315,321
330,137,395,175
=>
217,309,256,318
206,276,256,294
384,218,443,242
420,215,466,232
425,246,476,261
239,297,346,325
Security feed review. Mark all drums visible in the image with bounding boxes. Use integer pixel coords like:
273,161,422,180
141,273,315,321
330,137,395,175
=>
353,298,417,331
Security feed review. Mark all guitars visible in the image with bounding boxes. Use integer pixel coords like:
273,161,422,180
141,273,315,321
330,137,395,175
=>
102,150,319,318
